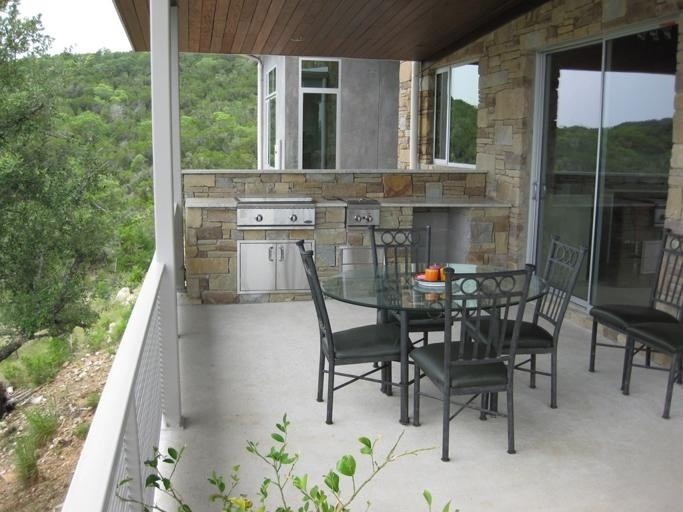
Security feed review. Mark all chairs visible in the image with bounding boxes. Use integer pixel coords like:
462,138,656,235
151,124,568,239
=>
620,321,682,419
408,260,537,462
296,238,413,426
365,223,456,398
462,233,589,423
587,228,682,373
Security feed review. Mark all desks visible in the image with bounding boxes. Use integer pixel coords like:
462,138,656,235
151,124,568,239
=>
376,195,512,281
319,261,551,425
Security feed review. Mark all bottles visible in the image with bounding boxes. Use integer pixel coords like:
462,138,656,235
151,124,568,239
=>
424,262,455,281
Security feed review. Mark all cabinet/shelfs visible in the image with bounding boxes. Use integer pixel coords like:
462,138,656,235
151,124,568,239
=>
233,239,316,295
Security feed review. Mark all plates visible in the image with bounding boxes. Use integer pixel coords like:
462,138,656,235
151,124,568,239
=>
412,284,460,293
413,274,461,287
